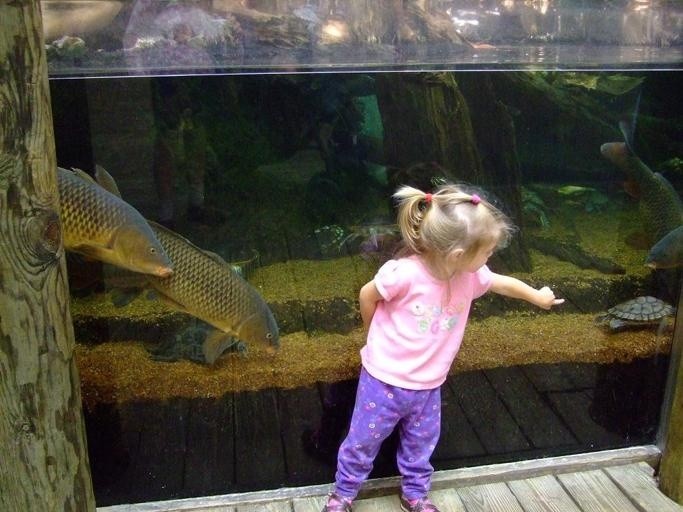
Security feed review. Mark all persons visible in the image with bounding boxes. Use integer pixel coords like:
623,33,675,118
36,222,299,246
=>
321,183,565,512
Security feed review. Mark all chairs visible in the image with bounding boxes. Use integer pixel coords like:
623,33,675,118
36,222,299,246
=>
321,491,352,511
400,493,441,512
